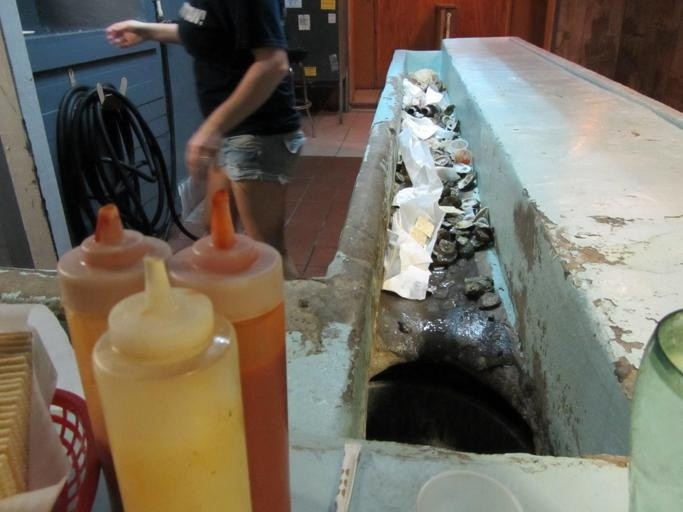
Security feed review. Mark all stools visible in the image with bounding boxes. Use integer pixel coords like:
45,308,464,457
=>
286,46,318,140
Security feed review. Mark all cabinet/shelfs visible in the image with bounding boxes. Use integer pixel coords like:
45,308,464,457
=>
278,0,349,125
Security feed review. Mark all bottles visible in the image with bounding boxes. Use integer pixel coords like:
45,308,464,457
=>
166,185,290,510
52,203,179,511
92,255,256,511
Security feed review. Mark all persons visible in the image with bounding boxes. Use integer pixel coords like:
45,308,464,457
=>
106,0,302,281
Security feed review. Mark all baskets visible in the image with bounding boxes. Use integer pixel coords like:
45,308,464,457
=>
48,388,100,512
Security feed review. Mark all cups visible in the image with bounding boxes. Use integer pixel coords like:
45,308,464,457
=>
626,308,682,511
413,469,520,511
